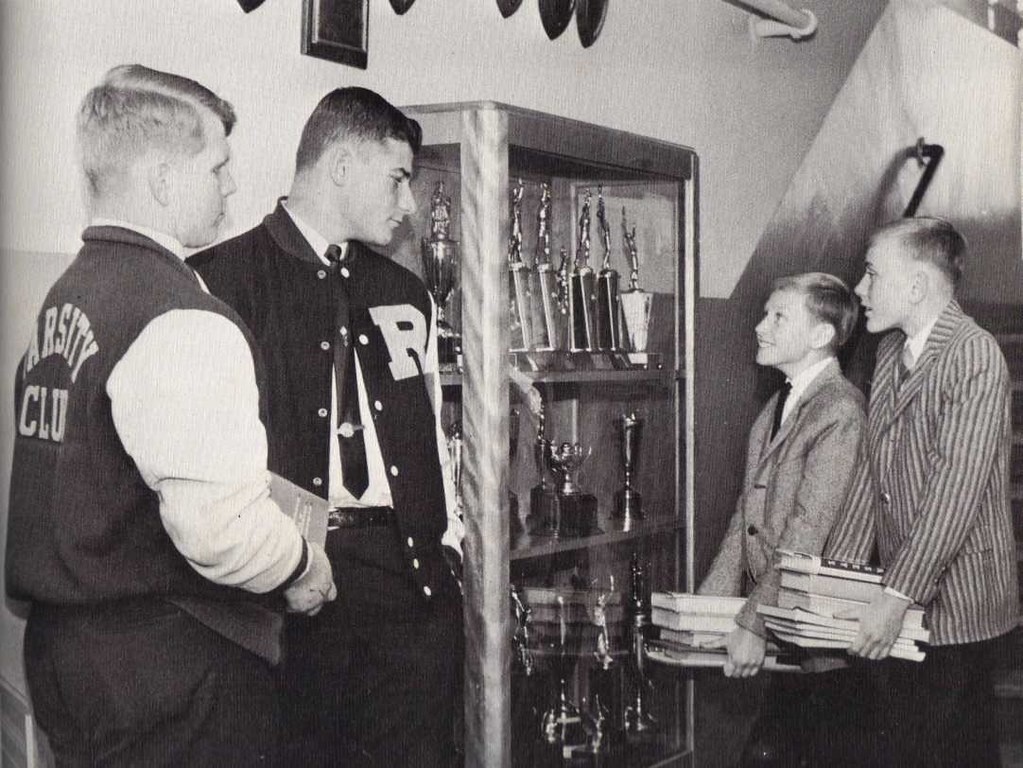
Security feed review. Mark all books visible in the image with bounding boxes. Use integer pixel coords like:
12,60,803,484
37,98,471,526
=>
641,548,929,669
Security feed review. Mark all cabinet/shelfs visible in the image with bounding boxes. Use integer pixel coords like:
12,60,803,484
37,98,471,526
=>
368,100,700,768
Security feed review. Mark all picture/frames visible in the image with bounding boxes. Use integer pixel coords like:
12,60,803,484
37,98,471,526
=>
301,0,369,69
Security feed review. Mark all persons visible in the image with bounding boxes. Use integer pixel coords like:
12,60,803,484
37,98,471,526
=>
693,215,1019,768
4,64,467,768
420,176,664,768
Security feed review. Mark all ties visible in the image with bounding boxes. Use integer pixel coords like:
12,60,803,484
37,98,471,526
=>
770,381,792,442
323,244,369,500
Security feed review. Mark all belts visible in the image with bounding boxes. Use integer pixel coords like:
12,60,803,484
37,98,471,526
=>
327,503,397,533
